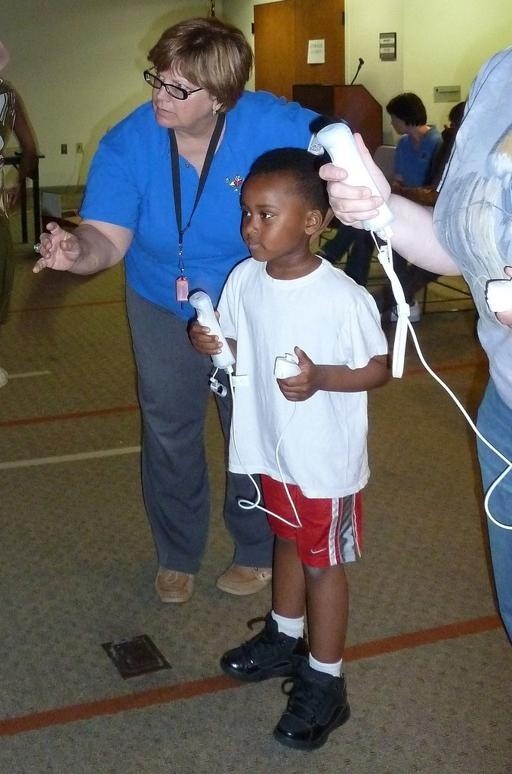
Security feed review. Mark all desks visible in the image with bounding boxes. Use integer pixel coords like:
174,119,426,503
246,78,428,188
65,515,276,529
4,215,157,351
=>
0,143,48,252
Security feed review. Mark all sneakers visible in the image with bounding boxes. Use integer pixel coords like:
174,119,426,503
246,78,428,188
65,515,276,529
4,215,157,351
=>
273,670,353,751
221,613,310,682
380,299,421,324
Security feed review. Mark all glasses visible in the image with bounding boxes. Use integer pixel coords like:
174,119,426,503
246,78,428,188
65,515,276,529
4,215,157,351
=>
143,66,205,101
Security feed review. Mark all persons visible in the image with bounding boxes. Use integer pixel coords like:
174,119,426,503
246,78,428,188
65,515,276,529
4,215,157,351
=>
316,48,512,645
192,142,386,748
371,98,471,329
319,90,443,291
1,72,40,392
33,15,337,603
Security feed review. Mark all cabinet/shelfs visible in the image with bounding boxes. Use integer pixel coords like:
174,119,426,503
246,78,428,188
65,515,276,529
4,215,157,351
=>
250,0,343,107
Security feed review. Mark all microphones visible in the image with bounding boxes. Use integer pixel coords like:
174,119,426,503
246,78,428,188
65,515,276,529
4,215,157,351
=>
351,58,364,84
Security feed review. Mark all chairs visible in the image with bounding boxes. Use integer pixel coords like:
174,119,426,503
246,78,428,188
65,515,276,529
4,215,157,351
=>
314,144,397,270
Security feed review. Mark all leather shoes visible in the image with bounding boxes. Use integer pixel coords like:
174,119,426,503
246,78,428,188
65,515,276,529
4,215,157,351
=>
155,563,195,606
216,561,273,597
